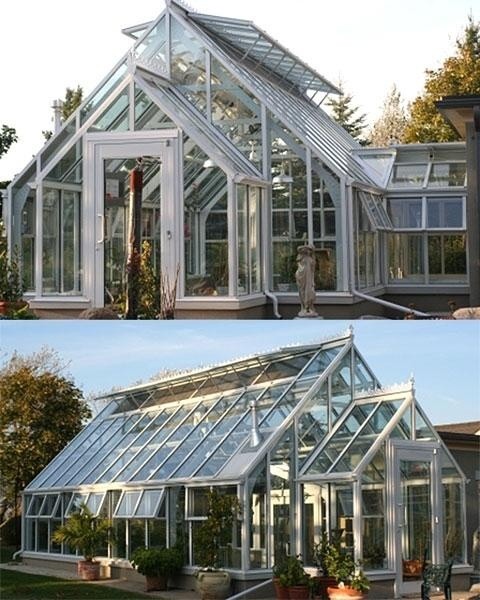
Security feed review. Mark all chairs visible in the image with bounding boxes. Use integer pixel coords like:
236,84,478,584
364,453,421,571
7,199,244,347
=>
418,559,453,599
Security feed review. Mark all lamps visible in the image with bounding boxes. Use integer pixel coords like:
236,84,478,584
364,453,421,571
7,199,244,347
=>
203,125,293,198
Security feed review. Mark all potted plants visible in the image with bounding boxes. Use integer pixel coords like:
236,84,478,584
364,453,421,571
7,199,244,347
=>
129,545,186,593
271,529,371,600
186,486,243,598
0,223,29,318
208,231,298,298
49,507,121,582
